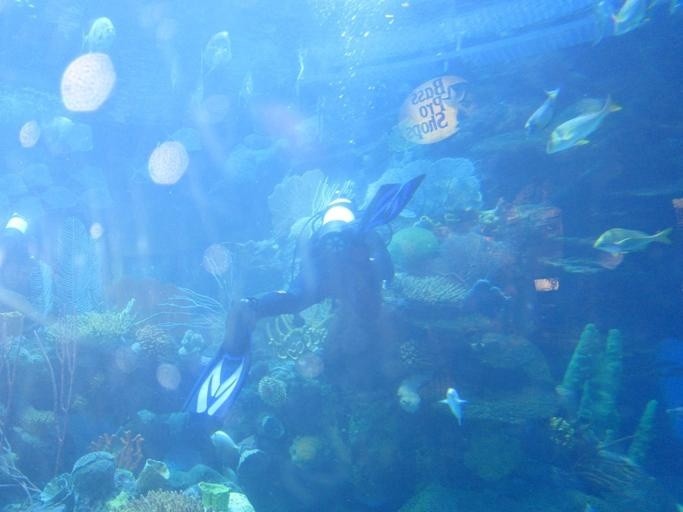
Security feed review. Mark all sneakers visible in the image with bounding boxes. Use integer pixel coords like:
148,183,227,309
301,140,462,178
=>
224,296,254,357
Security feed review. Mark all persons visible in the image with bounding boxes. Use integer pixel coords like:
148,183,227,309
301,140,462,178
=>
211,186,393,386
